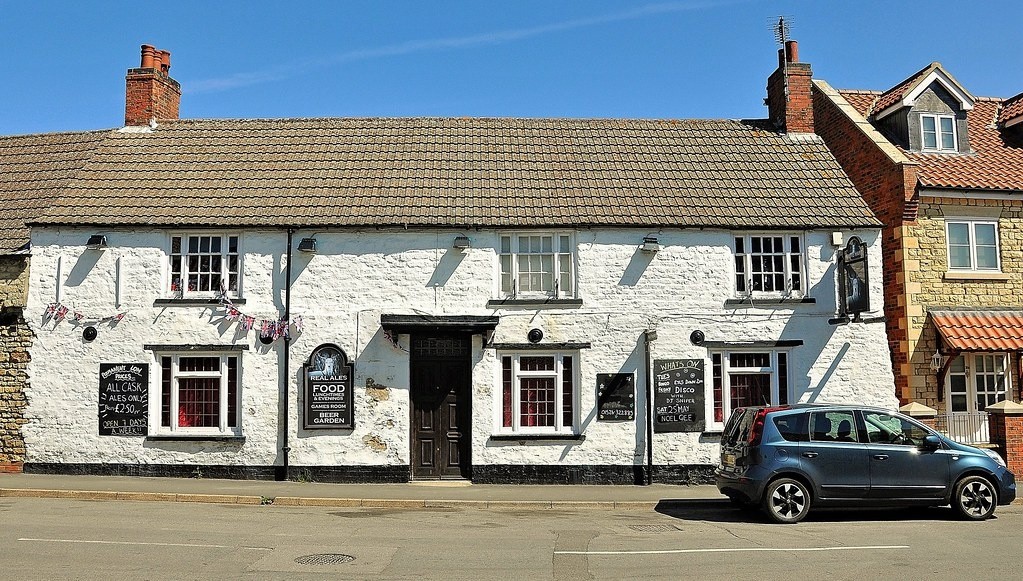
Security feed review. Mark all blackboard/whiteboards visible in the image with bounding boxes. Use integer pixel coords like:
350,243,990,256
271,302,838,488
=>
302,342,355,431
99,363,149,437
653,359,706,433
596,372,636,421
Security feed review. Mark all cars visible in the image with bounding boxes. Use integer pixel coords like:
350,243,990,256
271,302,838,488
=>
714,402,1017,525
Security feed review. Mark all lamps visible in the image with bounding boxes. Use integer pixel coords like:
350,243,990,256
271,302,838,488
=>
85,235,108,247
299,238,316,252
452,237,470,249
930,348,944,373
641,237,659,252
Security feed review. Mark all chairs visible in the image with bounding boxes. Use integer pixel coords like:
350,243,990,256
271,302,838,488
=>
814,417,835,441
835,420,856,442
